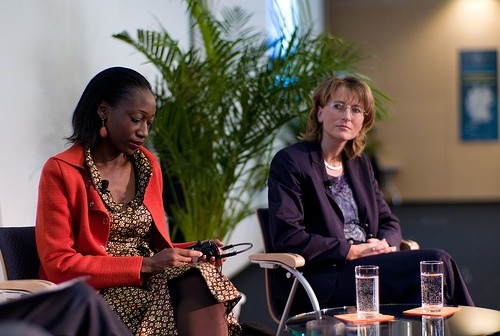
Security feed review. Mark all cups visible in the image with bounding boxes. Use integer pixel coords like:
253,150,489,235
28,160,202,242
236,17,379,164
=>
421,261,444,313
357,321,379,336
354,264,379,319
421,314,444,336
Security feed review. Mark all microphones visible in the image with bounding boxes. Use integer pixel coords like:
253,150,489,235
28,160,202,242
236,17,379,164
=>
100,180,109,193
327,179,332,190
284,306,357,326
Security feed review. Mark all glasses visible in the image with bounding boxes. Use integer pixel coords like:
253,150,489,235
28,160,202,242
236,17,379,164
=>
326,102,369,117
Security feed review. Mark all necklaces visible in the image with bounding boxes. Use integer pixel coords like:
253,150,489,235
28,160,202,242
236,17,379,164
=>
325,161,342,171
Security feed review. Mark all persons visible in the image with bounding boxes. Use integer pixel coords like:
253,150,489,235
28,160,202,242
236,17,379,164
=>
35,67,242,336
268,77,475,315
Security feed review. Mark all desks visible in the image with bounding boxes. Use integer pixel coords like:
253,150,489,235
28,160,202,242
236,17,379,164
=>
285,304,500,336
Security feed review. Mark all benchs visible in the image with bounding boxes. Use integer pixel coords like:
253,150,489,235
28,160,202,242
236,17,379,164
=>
248,207,500,336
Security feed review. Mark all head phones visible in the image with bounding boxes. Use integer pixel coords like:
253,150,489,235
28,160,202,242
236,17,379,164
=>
196,239,253,261
